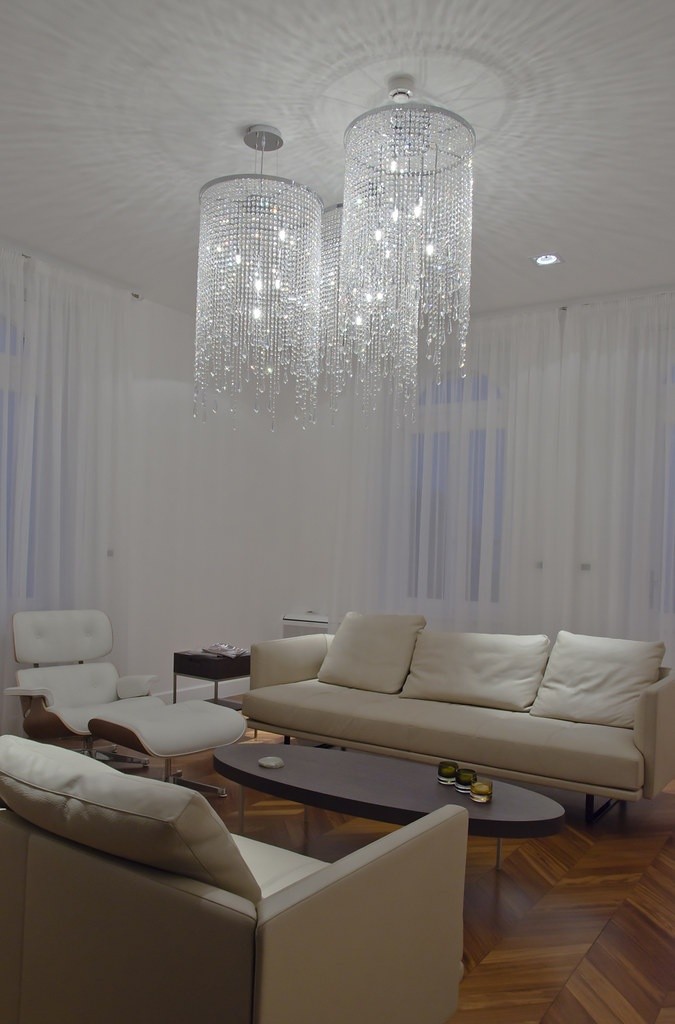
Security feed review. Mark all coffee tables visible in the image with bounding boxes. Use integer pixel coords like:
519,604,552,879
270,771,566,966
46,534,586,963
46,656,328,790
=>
211,745,566,873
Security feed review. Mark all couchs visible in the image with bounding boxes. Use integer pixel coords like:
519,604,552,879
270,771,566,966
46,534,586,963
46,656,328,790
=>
240,633,674,821
1,802,468,1024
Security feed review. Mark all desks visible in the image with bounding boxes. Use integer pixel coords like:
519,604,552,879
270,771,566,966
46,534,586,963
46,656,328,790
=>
172,650,250,706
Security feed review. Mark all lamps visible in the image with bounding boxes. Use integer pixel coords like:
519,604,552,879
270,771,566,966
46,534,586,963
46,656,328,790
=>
191,82,479,415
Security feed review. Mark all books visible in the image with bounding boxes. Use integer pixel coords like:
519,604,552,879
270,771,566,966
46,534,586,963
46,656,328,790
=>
201,641,247,660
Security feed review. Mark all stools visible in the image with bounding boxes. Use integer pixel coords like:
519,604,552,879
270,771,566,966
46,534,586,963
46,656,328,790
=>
87,699,248,797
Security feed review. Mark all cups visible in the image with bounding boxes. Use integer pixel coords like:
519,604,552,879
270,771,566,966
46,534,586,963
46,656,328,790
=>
470,776,493,803
437,760,459,785
455,767,476,794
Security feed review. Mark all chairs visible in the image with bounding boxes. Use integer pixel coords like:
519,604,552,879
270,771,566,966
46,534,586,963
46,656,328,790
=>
4,611,166,767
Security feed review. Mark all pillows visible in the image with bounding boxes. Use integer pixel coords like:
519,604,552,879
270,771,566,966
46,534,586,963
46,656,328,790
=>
1,728,264,902
316,610,426,691
400,629,553,713
530,630,665,729
